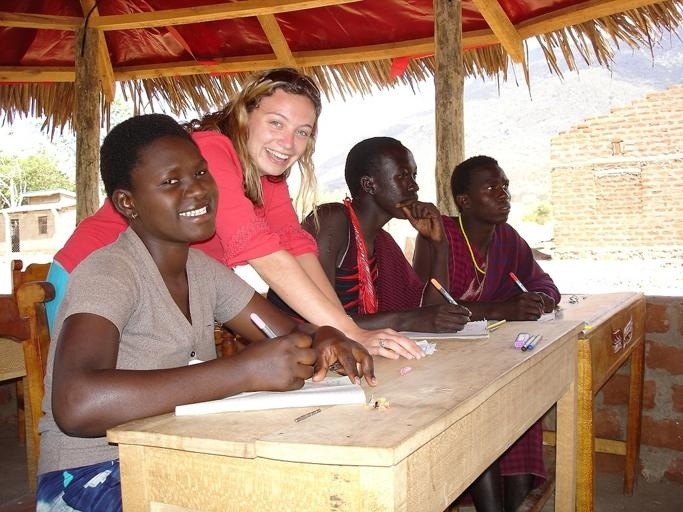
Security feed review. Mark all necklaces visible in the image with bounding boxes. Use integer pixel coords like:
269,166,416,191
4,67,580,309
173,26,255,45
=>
458,211,488,276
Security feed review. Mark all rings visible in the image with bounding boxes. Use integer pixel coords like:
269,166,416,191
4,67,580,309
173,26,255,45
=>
379,339,386,346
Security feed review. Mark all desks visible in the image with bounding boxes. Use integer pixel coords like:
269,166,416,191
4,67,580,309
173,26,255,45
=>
105,319,588,512
500,291,649,512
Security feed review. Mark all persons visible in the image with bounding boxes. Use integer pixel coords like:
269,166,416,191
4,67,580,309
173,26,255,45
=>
36,114,378,511
47,67,426,362
411,155,561,511
266,137,504,510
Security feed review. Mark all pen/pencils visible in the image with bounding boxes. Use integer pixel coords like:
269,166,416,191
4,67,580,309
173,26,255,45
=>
485,319,506,330
250,313,277,339
431,278,458,305
509,272,529,293
295,407,321,423
521,335,542,351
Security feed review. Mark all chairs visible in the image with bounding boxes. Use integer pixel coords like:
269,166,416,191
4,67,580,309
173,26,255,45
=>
0,257,245,512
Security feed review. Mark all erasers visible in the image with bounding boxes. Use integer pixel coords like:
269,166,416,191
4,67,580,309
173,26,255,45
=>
514,333,530,348
